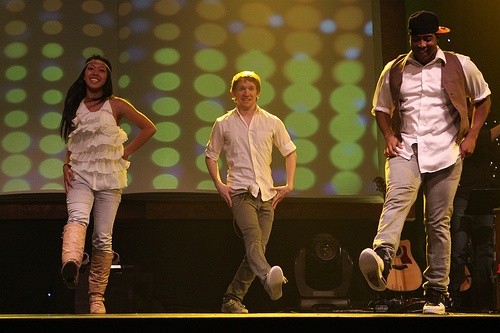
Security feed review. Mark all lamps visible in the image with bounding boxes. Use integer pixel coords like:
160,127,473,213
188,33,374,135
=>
295,233,354,310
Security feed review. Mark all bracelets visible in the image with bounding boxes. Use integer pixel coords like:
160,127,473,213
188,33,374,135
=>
63,164,71,168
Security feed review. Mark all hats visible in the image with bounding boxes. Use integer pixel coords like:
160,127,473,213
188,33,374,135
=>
407,10,450,34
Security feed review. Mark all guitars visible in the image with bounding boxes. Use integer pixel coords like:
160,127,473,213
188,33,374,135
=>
373,176,419,293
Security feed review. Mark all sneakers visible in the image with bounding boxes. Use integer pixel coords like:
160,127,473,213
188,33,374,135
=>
359,248,392,292
220,299,249,314
423,297,446,315
264,266,288,300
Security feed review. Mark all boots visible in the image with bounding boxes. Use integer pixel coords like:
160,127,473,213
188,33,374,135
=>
88,248,114,314
61,223,87,290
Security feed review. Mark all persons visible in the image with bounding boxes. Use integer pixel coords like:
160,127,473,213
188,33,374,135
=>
204,70,297,314
58,53,157,314
358,9,492,315
